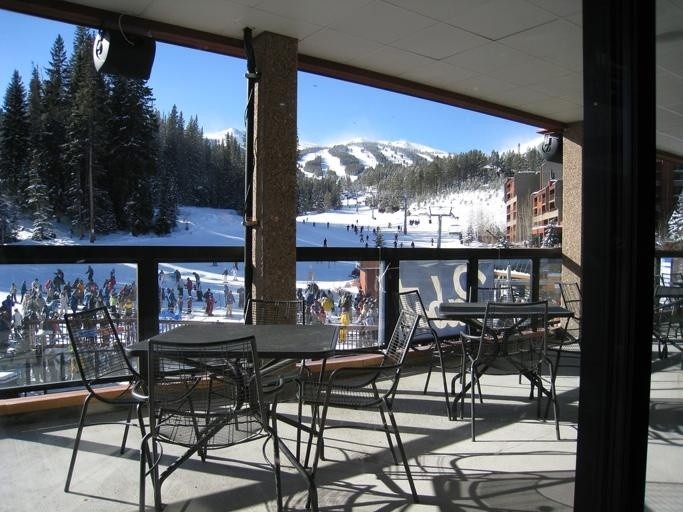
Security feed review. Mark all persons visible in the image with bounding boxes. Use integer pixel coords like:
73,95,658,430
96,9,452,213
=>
296,281,378,346
0,261,243,355
300,214,435,248
